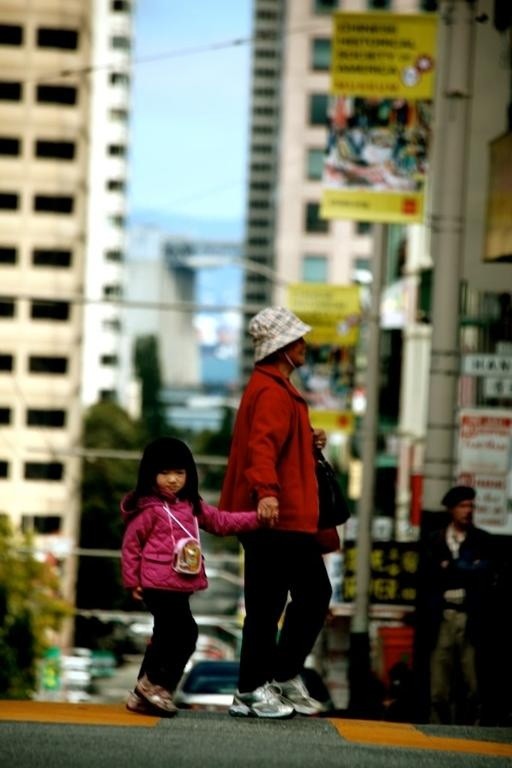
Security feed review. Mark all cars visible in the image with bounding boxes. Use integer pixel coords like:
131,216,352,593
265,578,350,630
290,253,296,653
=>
306,669,334,710
174,660,240,712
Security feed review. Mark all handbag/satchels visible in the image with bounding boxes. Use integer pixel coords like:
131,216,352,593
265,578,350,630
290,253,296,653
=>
312,434,351,530
171,537,202,576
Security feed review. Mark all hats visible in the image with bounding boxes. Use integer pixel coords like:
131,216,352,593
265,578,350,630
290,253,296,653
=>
441,485,475,505
247,305,312,364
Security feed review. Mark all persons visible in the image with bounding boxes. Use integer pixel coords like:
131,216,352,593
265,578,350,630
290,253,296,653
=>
120,437,279,717
217,302,350,720
421,486,497,726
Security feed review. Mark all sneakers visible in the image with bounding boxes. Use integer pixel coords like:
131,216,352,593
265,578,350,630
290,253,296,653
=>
271,675,324,715
126,672,179,719
228,681,297,720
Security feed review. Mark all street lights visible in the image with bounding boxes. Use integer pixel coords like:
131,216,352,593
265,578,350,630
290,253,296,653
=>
185,255,289,285
